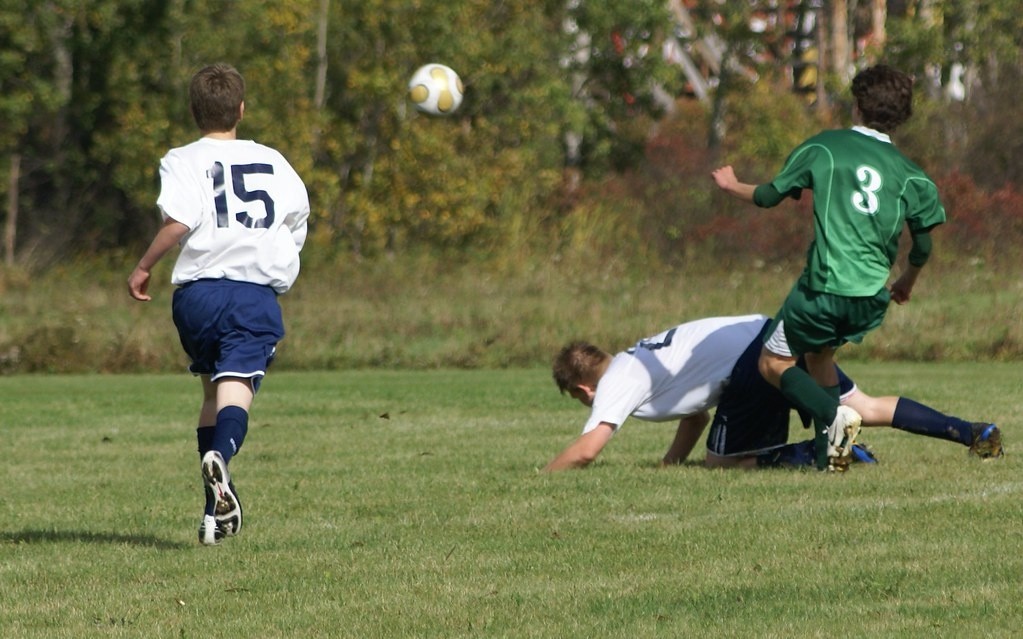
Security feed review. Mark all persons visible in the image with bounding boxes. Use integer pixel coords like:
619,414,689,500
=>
711,63,947,473
539,312,1004,472
126,63,310,545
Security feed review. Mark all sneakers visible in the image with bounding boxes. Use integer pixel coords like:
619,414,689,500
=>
823,405,862,474
201,450,243,536
970,423,1004,458
852,445,876,465
198,513,224,545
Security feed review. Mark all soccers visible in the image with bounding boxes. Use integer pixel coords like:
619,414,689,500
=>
408,61,466,118
